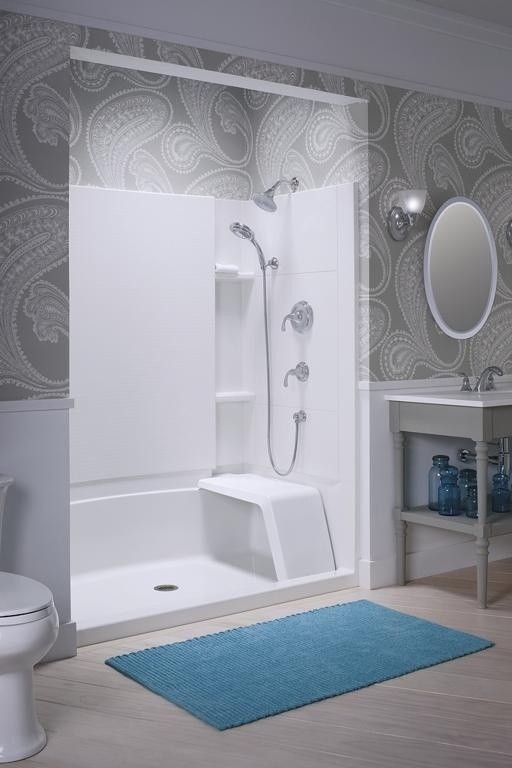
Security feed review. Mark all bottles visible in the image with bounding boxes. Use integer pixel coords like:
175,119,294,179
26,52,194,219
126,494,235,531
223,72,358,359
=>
427,454,486,519
492,473,511,513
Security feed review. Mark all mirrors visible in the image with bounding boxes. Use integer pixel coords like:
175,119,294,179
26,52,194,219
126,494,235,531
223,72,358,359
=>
422,196,498,341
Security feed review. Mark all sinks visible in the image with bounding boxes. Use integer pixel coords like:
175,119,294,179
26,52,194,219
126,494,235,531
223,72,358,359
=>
479,391,512,393
384,389,512,409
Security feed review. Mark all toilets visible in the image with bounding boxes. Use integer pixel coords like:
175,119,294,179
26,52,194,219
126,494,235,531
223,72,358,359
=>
0,473,60,764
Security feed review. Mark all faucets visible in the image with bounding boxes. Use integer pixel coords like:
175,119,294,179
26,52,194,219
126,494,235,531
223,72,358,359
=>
474,366,503,392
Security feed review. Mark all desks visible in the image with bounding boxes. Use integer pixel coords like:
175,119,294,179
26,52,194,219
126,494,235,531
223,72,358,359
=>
383,388,510,609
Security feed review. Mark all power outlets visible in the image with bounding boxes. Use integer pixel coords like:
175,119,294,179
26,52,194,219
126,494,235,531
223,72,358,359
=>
387,190,427,240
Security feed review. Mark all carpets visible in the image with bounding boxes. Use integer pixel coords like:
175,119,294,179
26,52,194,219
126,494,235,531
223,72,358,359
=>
106,601,494,731
